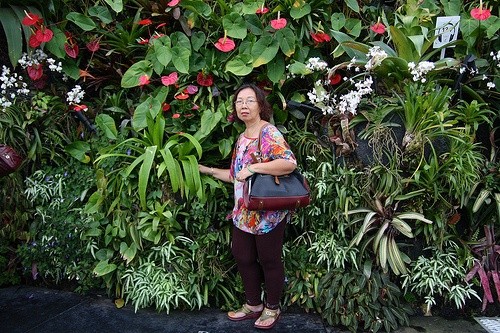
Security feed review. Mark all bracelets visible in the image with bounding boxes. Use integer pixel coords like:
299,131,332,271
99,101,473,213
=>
247,165,254,174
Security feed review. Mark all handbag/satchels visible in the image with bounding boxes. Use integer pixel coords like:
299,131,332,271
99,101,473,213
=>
244,123,310,210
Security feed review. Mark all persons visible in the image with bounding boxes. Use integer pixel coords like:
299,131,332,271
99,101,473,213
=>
198,84,297,329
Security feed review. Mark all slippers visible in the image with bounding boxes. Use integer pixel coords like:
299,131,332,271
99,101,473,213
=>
228,305,263,320
254,307,281,328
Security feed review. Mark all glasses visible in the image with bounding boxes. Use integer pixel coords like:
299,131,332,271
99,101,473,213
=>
234,98,257,105
